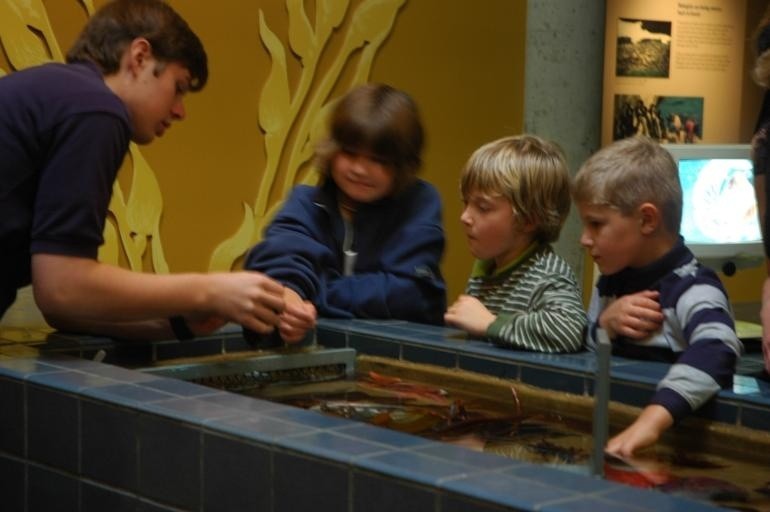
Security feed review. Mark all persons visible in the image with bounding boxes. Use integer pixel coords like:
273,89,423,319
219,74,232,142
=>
0,1,286,336
442,132,589,355
242,82,448,350
569,135,745,458
747,14,770,376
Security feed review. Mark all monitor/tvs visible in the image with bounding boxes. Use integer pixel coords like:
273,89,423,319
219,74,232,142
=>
661,142,768,260
